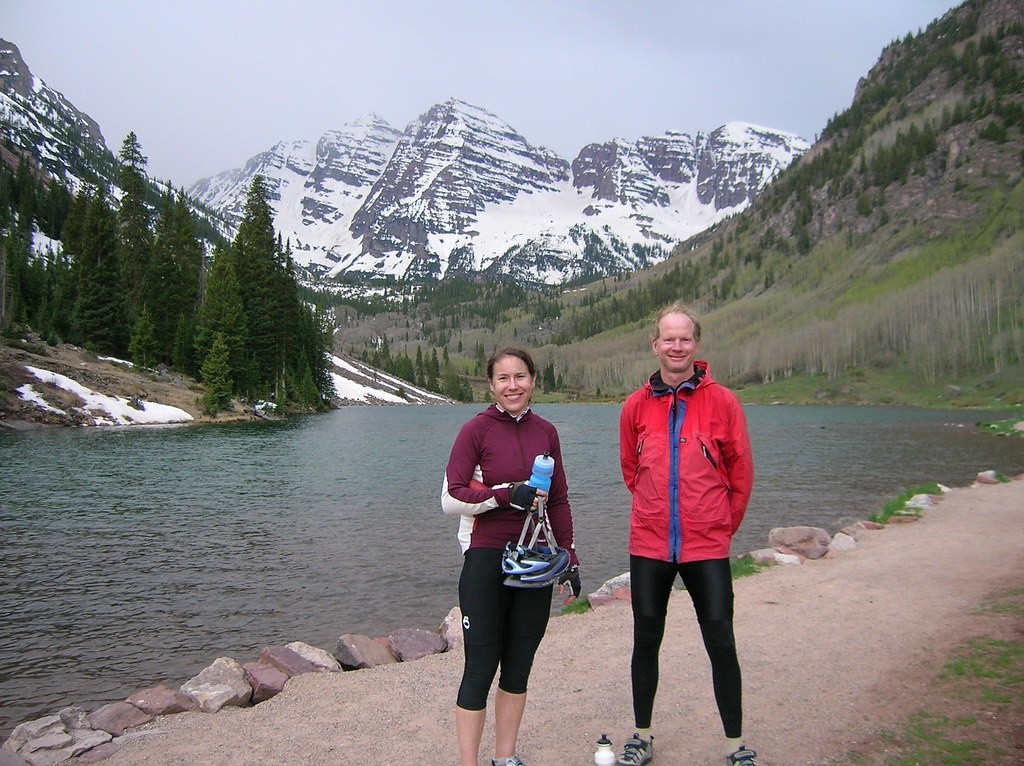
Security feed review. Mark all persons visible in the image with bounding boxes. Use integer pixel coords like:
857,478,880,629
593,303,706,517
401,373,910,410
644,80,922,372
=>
441,347,582,766
618,305,758,766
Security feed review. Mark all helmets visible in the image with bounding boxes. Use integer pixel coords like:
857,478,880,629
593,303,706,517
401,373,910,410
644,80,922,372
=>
502,540,571,588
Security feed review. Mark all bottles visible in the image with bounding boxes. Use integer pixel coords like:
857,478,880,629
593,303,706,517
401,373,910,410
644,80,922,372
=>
529,451,554,493
594,734,616,766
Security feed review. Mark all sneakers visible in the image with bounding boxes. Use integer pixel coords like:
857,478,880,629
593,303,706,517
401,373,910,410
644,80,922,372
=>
492,756,526,766
727,744,759,766
618,733,654,766
